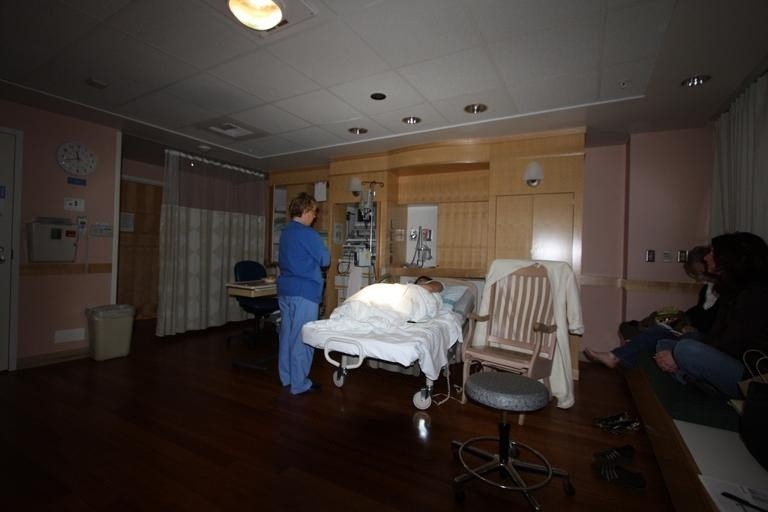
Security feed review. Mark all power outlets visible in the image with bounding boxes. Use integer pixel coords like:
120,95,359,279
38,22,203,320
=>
64,197,85,212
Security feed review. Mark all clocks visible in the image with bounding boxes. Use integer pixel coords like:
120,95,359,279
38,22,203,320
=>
57,142,98,177
227,0,284,32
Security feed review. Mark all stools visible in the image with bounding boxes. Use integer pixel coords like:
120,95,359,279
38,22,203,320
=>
450,371,575,512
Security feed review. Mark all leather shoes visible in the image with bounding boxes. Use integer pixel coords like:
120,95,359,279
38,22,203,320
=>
288,382,320,396
607,419,641,436
592,412,630,428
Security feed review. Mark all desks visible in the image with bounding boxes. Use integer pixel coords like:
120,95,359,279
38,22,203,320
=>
225,280,278,371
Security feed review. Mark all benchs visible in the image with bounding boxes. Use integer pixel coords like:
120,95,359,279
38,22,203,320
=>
617,325,768,512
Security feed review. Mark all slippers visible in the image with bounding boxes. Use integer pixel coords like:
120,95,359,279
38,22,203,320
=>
591,463,643,488
593,445,634,461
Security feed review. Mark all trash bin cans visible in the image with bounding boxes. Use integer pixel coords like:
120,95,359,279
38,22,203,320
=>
85,304,135,361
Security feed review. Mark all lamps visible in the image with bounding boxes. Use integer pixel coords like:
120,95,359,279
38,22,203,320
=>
520,160,544,187
347,176,361,196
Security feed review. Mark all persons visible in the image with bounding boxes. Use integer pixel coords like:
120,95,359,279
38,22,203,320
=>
653,231,768,400
414,276,446,292
276,193,331,397
583,247,720,369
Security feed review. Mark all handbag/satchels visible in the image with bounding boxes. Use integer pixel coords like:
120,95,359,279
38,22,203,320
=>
740,349,768,473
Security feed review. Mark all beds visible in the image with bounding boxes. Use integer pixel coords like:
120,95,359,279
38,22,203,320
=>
301,276,478,411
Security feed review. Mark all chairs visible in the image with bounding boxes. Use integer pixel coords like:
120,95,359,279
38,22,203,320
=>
224,260,280,350
459,257,584,426
